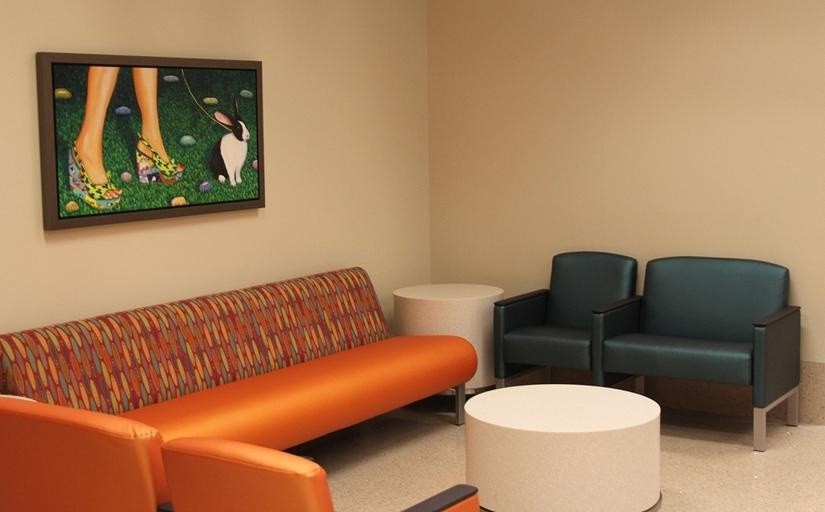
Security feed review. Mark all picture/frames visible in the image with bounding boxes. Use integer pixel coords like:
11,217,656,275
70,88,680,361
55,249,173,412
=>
35,51,265,232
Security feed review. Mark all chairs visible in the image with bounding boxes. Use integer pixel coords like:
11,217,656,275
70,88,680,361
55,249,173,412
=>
2,392,160,510
162,431,481,512
495,251,801,451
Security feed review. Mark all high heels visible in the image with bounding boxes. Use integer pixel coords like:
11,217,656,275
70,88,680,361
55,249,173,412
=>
135,136,184,184
68,147,122,209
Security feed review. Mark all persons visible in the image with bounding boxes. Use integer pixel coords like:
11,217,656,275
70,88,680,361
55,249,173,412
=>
69,67,184,209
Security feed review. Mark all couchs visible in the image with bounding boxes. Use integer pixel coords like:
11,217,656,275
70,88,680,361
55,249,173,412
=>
0,266,477,453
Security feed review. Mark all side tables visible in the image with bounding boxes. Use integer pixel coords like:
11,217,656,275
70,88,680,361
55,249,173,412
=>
388,281,506,390
